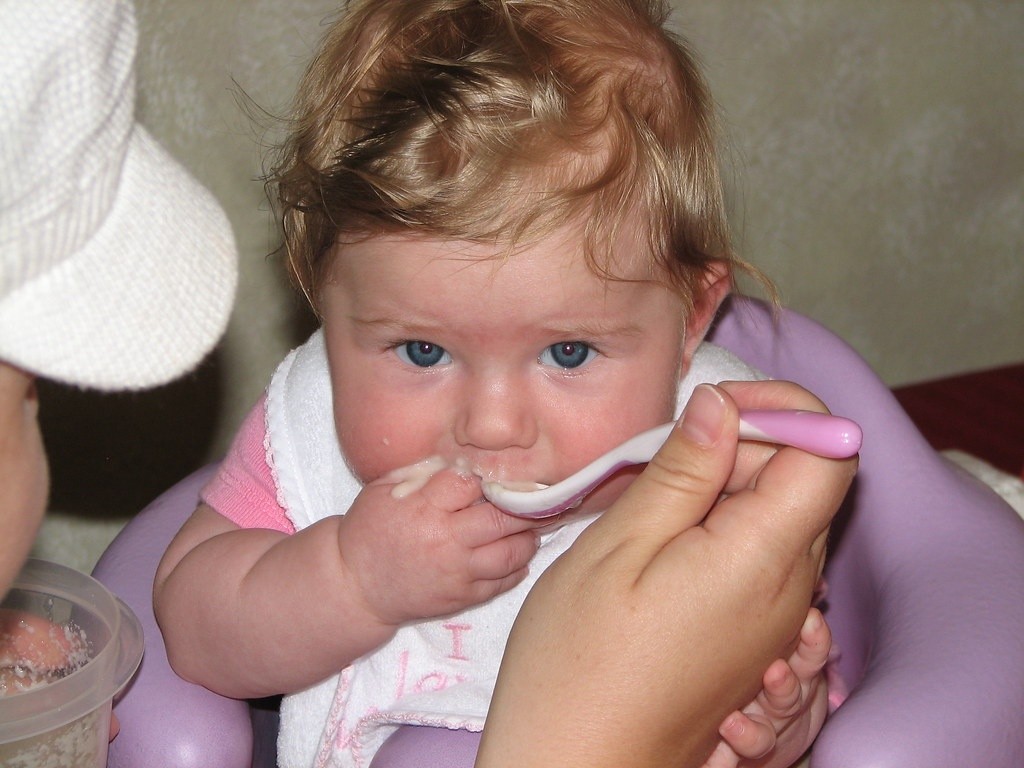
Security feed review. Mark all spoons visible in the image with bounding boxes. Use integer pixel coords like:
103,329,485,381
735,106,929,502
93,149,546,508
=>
479,410,865,519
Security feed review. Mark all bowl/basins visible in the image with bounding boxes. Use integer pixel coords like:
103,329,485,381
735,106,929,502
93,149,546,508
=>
1,558,146,768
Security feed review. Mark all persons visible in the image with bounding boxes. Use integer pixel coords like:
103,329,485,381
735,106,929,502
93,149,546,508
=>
151,0,831,768
0,0,858,768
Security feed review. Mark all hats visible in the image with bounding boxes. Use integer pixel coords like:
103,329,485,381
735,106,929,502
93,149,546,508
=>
0,0,239,391
